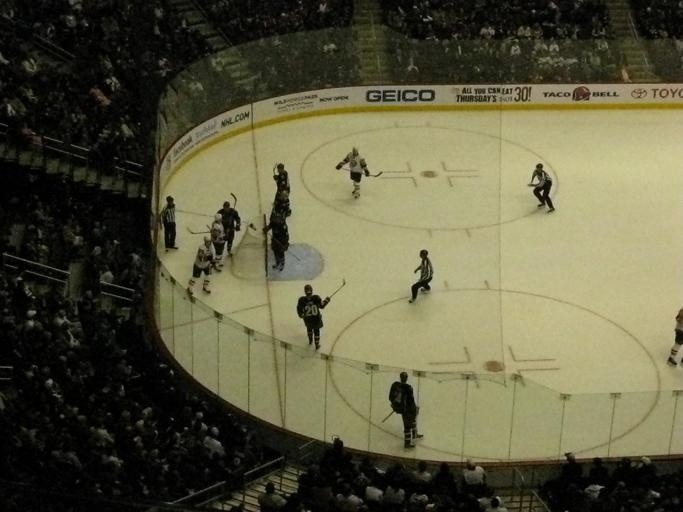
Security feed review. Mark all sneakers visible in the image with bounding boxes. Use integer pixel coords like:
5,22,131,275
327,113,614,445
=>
405,442,415,447
316,345,319,349
187,251,234,294
409,297,415,303
413,434,423,438
421,288,430,291
272,262,284,271
668,356,677,365
538,203,545,206
352,190,361,199
548,209,555,212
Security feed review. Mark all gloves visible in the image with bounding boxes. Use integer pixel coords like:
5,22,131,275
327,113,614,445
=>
323,297,330,304
336,164,342,169
236,225,240,231
365,170,370,176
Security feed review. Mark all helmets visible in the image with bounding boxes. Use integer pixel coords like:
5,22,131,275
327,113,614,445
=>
305,285,312,293
204,201,229,246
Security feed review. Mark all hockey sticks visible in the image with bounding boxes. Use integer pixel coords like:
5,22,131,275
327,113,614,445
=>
340,167,383,177
227,193,237,236
211,258,222,272
249,222,302,261
329,277,345,298
273,163,277,176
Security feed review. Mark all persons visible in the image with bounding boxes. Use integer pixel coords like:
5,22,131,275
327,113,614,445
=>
210,1,683,96
336,146,370,200
258,438,681,511
389,371,423,448
0,1,296,511
528,163,556,214
297,285,331,351
409,250,434,303
667,310,682,367
